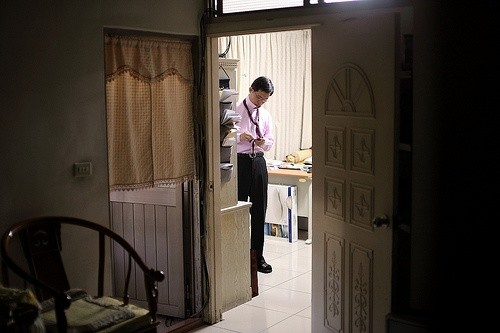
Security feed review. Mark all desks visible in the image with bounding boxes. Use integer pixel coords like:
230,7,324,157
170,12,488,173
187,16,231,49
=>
266,167,312,244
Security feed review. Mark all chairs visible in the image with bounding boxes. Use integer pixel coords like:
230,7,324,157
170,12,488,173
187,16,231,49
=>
0,214,166,333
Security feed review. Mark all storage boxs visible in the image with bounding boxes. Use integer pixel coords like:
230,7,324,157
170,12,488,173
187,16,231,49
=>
264,184,298,243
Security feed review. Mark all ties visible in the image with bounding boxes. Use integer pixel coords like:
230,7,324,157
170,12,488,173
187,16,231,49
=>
243,98,263,159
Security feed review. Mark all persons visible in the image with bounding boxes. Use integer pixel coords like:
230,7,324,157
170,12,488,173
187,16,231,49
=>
235,76,274,273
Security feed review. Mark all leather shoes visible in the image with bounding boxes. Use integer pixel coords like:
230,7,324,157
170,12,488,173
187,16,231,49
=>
257,262,272,273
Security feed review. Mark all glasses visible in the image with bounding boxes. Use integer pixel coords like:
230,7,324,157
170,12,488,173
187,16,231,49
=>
251,87,268,102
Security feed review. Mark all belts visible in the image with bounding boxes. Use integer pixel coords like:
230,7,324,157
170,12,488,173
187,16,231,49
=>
237,152,264,158
